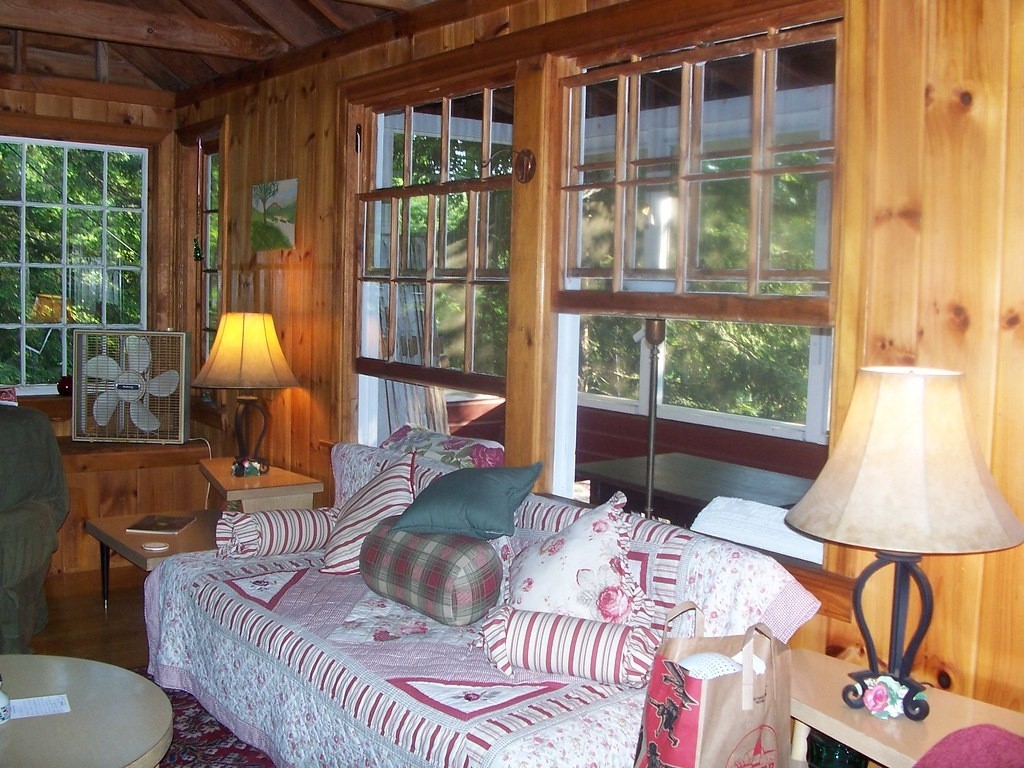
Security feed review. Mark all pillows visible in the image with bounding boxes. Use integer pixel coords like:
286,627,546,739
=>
509,528,656,604
360,515,503,626
478,603,664,691
320,451,416,574
214,506,340,558
388,461,543,542
500,490,656,629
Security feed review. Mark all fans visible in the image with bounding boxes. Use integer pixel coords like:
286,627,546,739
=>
71,330,191,444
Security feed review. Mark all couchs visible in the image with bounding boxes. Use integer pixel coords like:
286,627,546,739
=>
145,441,829,768
0,406,71,654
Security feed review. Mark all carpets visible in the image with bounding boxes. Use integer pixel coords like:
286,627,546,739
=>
130,667,275,768
0,654,173,768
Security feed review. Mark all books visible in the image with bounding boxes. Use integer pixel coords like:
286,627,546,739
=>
126,515,197,535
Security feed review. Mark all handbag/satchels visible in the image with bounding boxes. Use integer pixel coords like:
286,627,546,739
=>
633,600,791,768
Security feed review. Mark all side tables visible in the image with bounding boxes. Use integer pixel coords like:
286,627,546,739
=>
83,458,325,609
190,311,301,475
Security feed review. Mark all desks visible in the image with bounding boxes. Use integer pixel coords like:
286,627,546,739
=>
786,647,1023,768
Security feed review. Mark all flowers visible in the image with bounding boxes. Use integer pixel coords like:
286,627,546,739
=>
860,674,906,718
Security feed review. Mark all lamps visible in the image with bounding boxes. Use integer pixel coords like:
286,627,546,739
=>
782,365,1024,723
27,294,73,323
